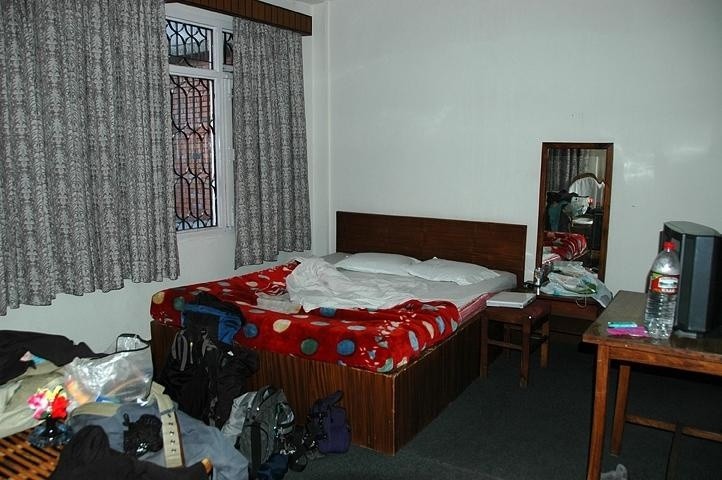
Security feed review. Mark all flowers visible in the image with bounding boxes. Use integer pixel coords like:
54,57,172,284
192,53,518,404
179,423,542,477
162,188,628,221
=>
26,384,70,420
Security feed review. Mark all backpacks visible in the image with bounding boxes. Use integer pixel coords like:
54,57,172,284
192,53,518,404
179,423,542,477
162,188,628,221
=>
152,292,259,427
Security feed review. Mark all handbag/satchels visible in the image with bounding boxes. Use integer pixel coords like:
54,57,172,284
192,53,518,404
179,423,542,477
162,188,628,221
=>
221,385,294,468
305,392,352,453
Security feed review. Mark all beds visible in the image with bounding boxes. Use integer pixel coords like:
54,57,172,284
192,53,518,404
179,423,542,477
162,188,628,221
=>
150,208,527,455
542,230,590,273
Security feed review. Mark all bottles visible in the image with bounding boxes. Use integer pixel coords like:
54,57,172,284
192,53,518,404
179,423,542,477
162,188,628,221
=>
644,241,681,340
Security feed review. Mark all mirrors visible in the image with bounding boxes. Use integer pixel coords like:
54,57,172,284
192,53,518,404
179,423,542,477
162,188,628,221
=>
534,141,613,283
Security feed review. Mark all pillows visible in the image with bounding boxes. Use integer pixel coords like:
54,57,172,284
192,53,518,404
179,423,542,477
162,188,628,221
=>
334,252,422,277
407,257,499,285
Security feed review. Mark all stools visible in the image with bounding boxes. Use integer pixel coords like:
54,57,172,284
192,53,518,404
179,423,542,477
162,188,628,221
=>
570,224,594,251
481,300,551,388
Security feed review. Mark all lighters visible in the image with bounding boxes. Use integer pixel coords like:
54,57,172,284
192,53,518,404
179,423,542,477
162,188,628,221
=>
606,321,637,328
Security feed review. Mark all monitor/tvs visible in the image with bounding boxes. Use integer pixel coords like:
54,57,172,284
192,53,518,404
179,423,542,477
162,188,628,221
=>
658,221,722,339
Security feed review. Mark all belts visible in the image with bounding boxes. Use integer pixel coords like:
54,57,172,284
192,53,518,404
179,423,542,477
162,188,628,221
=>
152,390,183,469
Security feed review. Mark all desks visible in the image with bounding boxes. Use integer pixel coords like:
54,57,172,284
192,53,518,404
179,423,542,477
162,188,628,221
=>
581,289,722,480
1,429,212,479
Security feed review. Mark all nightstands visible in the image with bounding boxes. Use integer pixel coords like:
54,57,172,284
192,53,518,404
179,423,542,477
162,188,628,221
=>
509,282,602,335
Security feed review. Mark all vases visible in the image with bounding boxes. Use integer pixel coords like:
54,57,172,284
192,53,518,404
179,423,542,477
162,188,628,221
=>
28,420,73,447
